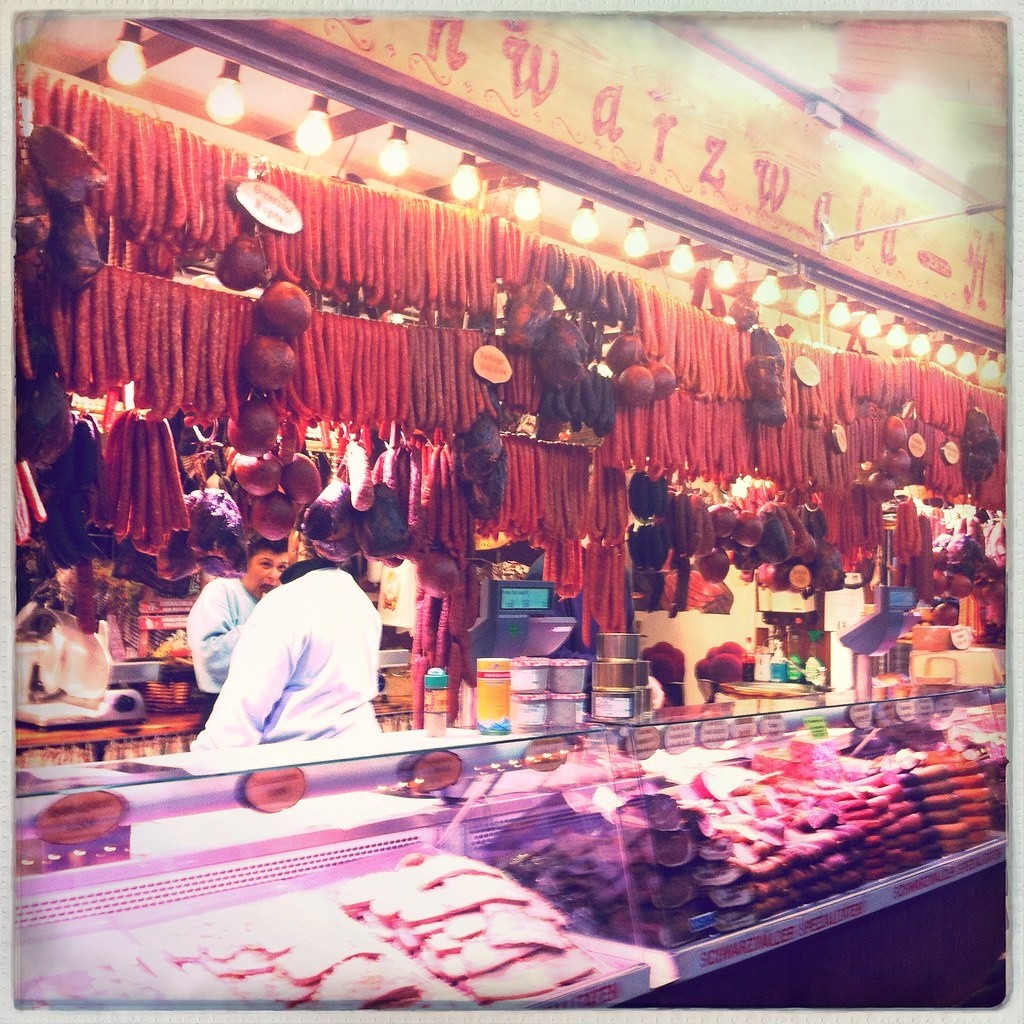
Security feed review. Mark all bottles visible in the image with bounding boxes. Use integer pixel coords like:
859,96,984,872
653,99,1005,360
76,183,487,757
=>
742,637,755,681
475,657,512,735
424,674,447,737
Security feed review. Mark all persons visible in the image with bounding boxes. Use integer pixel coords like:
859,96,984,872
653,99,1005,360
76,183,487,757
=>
186,533,383,756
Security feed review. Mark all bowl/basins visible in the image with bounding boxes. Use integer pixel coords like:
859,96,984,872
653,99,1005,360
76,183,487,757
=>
511,634,652,727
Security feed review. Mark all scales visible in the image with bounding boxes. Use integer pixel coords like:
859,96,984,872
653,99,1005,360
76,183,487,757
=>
836,511,923,658
466,578,576,679
15,662,162,732
